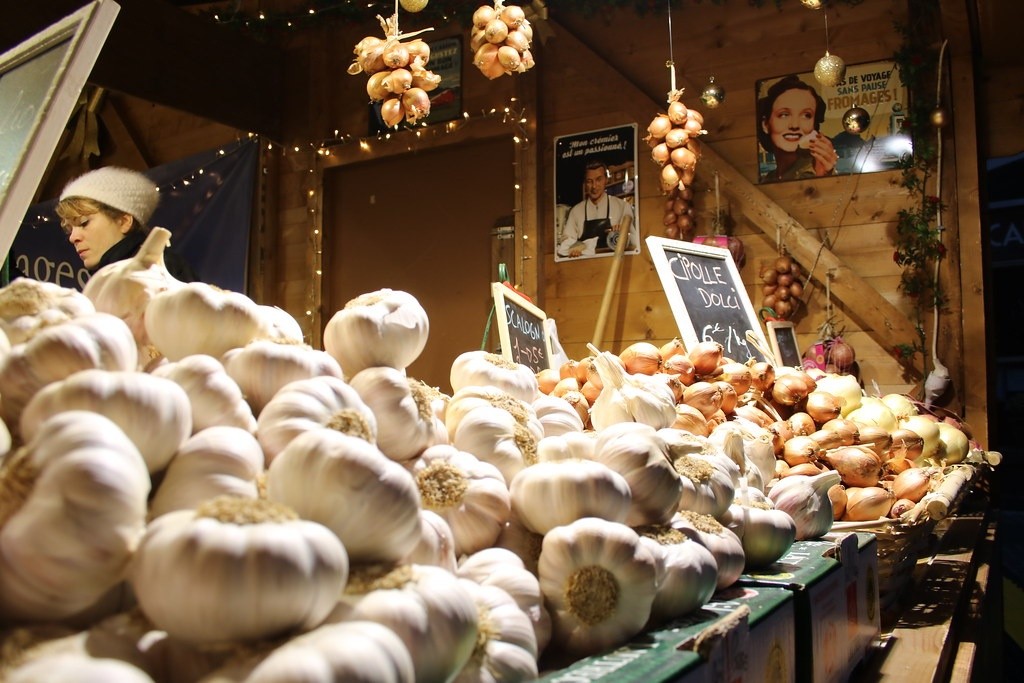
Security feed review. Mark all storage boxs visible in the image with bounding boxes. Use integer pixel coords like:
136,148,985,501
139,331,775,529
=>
533,586,796,683
737,532,882,683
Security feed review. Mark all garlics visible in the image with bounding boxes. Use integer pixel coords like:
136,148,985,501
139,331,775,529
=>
0,224,840,683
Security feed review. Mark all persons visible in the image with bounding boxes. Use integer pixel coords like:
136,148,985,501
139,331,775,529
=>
557,161,637,258
757,75,840,184
54,166,188,283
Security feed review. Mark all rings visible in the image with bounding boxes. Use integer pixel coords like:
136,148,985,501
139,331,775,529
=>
831,149,837,156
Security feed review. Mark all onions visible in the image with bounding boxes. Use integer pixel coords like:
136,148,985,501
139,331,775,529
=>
527,340,976,523
469,5,535,80
759,256,804,317
353,37,442,127
645,101,744,267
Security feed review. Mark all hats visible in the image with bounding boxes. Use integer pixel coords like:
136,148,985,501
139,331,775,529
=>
59,165,161,229
